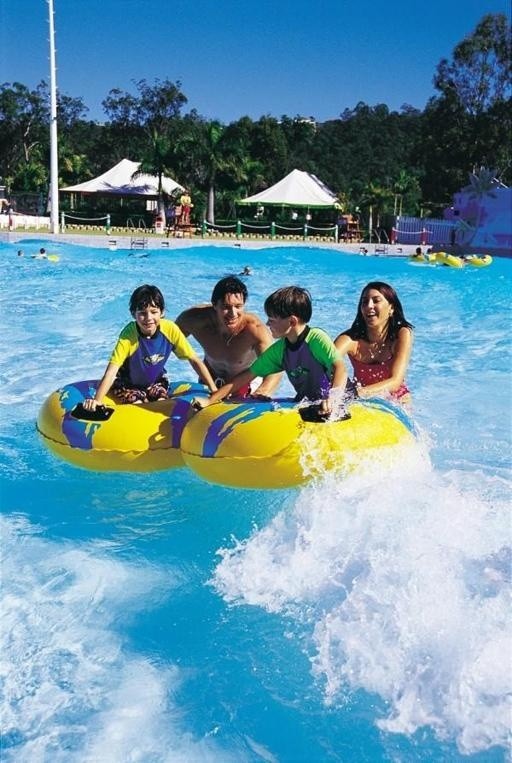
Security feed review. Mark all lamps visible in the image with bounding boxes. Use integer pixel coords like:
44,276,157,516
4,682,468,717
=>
291,205,312,221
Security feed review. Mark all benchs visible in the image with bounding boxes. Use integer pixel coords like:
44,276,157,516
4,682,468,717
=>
131,236,147,250
375,244,388,254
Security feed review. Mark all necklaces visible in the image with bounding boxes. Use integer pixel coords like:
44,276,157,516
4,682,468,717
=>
219,327,240,346
366,342,388,360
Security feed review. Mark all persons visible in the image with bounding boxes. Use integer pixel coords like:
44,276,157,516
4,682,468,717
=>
255,203,265,221
330,280,413,409
174,276,283,399
83,283,219,412
359,246,487,266
179,189,191,223
16,249,24,257
189,286,356,414
291,210,298,220
35,247,47,259
240,265,253,276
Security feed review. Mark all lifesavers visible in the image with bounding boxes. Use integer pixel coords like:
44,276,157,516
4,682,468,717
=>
181,398,421,487
36,380,213,474
412,253,491,267
